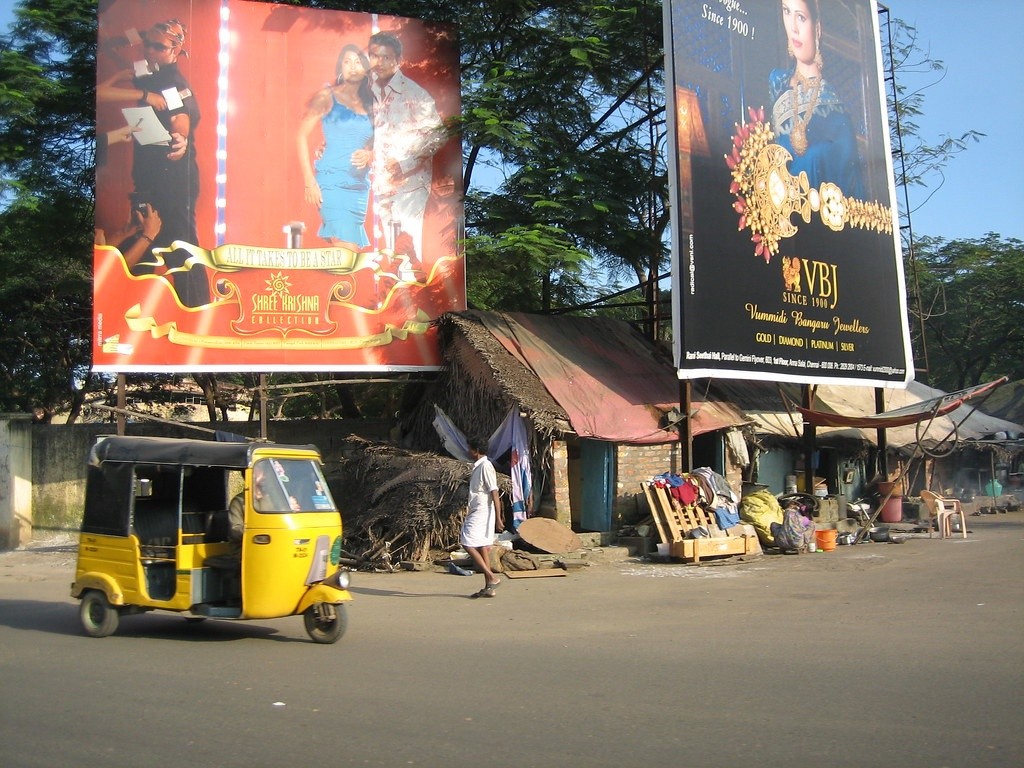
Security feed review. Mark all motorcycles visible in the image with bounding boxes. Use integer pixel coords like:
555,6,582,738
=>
71,436,354,643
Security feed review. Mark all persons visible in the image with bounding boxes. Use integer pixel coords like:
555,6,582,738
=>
314,30,447,262
229,464,276,556
770,502,815,554
95,17,213,307
299,44,372,252
764,0,870,216
459,436,506,598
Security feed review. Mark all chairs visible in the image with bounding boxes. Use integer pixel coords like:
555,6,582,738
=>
920,490,968,540
204,510,229,544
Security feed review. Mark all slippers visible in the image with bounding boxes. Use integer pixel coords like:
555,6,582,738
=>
480,579,503,595
470,589,496,598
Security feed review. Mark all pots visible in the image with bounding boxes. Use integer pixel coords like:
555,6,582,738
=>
877,481,901,497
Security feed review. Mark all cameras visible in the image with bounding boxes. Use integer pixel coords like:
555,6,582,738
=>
128,191,160,225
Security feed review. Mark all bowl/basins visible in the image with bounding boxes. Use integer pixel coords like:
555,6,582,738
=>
816,549,823,552
855,528,871,541
869,527,889,542
891,536,908,544
840,536,851,545
964,496,975,503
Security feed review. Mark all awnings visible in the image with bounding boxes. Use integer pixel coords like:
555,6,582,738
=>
798,377,1024,458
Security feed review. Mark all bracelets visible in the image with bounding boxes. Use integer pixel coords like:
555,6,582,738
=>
140,87,147,104
141,235,153,244
177,131,188,144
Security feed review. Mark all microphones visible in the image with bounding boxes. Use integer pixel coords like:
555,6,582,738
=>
114,28,147,46
130,60,159,78
161,87,192,110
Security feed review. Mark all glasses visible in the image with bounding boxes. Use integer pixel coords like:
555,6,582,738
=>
144,39,177,52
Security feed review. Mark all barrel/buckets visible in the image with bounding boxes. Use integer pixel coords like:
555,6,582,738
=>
815,529,837,550
809,543,815,552
879,497,903,523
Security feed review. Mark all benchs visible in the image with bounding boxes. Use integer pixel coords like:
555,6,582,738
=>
133,511,207,599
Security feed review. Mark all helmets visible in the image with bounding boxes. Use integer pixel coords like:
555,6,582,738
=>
254,466,267,487
